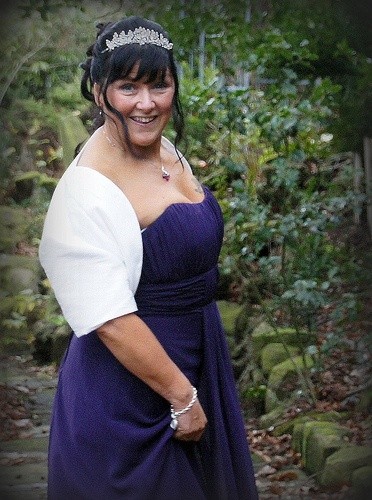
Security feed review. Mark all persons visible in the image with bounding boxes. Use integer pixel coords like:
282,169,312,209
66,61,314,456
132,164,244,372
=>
37,15,261,500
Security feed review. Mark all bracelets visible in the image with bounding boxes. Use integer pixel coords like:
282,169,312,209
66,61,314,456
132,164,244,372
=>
170,385,198,431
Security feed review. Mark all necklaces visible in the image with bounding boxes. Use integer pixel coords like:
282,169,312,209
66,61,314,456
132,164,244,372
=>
102,124,171,182
